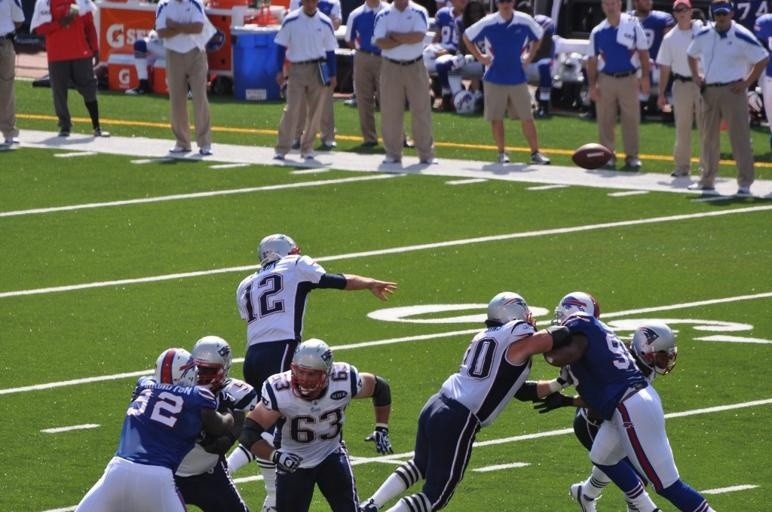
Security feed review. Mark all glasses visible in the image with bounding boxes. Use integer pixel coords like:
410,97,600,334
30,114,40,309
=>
716,11,728,16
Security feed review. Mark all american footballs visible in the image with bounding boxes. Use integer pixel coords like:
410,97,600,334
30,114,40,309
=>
544,335,587,366
572,143,611,168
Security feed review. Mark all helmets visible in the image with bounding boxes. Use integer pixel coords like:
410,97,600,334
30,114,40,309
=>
453,91,475,114
291,338,333,399
154,335,232,392
488,291,536,328
550,291,600,326
257,233,300,267
631,323,678,375
561,52,583,81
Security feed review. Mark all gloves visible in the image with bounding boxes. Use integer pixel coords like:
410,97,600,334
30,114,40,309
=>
270,450,303,474
557,364,573,388
365,422,394,455
532,391,574,413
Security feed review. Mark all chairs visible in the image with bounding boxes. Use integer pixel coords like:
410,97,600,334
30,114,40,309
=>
541,40,594,117
421,32,451,106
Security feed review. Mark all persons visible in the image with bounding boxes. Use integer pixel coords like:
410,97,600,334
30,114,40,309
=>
573,322,677,512
359,291,569,512
224,234,399,512
30,0,110,137
242,338,392,512
526,291,712,511
344,0,441,164
1,0,25,147
462,0,551,165
135,51,156,95
273,0,343,160
173,335,258,512
78,348,232,511
424,1,555,119
584,0,772,199
155,1,213,155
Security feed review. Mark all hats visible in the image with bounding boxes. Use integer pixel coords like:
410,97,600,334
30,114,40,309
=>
673,0,691,9
711,3,731,13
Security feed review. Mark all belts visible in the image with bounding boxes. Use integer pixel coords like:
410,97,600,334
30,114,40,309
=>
674,73,691,81
703,81,728,86
296,60,318,63
384,55,422,65
604,69,636,78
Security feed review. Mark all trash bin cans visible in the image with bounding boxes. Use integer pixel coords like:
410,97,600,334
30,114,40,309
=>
229,24,283,103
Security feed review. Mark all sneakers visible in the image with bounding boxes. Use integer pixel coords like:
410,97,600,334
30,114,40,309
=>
200,148,212,155
324,140,336,147
738,186,749,194
383,158,401,163
606,159,615,167
672,170,689,176
628,159,641,167
95,129,110,136
59,127,70,136
570,482,597,512
420,159,438,164
273,153,284,159
170,146,190,152
292,138,300,149
304,153,314,162
531,151,550,164
6,137,19,143
625,496,640,512
496,151,510,163
688,180,714,190
